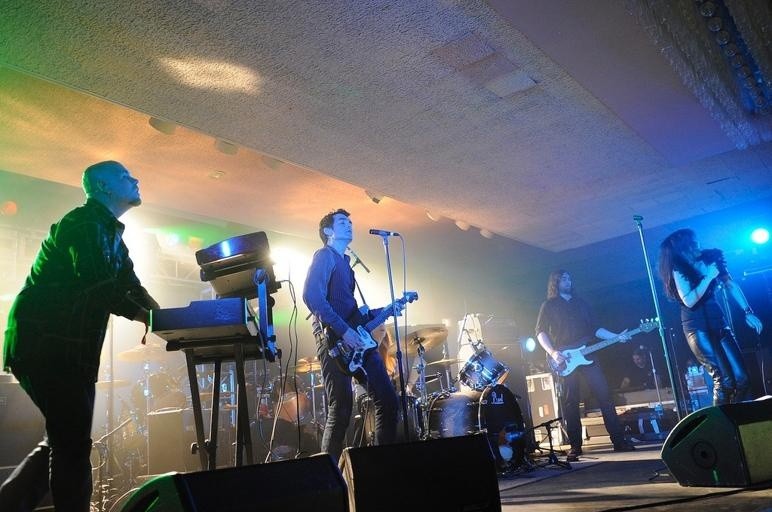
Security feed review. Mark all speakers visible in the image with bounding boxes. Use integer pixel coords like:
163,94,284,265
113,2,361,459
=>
115,454,351,512
659,400,771,486
146,406,231,476
344,430,501,512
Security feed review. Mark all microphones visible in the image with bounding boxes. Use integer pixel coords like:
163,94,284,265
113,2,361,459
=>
503,431,529,442
367,225,398,241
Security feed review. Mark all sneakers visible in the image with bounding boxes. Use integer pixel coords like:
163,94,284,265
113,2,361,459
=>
567,447,583,456
614,440,636,452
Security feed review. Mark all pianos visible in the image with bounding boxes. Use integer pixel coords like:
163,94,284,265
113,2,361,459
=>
195,231,278,300
150,298,272,364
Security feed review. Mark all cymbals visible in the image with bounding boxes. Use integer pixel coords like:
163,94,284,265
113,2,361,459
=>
403,324,448,354
292,356,321,372
115,348,176,360
95,379,129,386
424,358,461,367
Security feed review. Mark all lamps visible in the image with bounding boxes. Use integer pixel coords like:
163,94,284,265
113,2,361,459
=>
261,156,284,173
148,116,175,137
453,221,472,232
363,189,385,205
424,208,443,222
481,230,493,240
213,138,239,155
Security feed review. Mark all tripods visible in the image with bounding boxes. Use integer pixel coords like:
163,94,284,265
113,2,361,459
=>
532,451,570,470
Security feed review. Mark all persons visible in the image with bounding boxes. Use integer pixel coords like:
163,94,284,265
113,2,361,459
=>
620,348,661,389
659,228,762,406
350,324,413,421
535,268,636,455
0,161,159,512
302,208,405,467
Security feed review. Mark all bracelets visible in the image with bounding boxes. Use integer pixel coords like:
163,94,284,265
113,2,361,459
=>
744,306,754,315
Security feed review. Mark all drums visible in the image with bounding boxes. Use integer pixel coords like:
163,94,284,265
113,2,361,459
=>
273,371,310,421
456,351,510,393
424,384,527,475
129,371,186,423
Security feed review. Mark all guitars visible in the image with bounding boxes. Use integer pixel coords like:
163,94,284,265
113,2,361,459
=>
324,293,419,376
546,316,661,376
